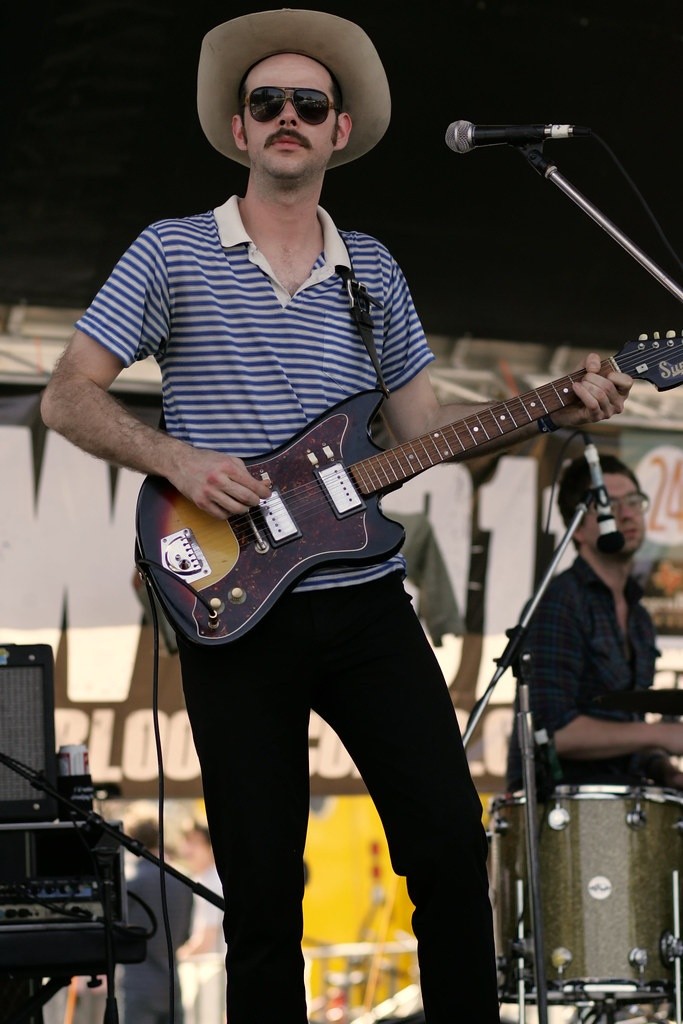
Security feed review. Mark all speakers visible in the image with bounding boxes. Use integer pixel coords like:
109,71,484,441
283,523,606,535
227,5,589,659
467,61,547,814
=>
0,644,59,823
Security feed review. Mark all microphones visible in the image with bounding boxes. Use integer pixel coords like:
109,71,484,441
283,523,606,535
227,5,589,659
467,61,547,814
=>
446,120,595,153
580,432,625,554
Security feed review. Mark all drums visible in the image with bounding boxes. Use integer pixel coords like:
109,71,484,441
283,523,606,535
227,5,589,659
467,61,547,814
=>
487,782,683,1004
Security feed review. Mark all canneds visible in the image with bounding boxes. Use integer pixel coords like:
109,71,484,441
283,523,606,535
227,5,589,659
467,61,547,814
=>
57,743,89,776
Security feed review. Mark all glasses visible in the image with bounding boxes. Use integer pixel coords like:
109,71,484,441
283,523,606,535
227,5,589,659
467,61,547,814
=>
241,86,338,125
610,492,651,515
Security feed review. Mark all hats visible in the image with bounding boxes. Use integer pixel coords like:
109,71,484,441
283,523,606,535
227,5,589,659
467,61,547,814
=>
182,818,211,845
197,9,391,171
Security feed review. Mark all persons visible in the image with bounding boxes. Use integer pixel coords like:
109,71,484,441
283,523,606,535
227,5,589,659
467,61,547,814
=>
38,8,636,1024
507,451,683,794
121,820,193,1024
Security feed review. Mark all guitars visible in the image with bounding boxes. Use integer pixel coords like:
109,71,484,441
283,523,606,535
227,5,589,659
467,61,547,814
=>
133,330,683,647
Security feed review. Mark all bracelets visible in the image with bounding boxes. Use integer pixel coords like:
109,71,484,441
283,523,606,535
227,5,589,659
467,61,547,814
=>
536,416,562,433
